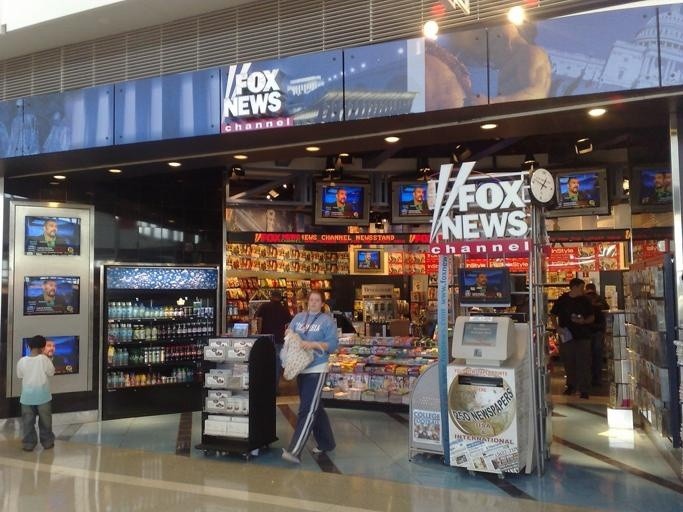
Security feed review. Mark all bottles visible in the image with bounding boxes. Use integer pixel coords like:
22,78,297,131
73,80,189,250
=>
227,302,239,316
159,322,216,339
108,323,158,343
172,363,208,383
108,301,184,317
108,344,208,366
193,307,214,319
162,376,177,383
107,371,161,387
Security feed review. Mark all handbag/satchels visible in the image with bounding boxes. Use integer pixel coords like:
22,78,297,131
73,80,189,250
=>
279,332,315,381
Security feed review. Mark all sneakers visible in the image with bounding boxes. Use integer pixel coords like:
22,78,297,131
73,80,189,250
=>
311,447,324,455
281,448,301,464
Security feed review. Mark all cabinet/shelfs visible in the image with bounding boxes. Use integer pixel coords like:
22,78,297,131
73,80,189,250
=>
319,331,441,406
547,284,571,357
195,333,278,462
98,264,219,422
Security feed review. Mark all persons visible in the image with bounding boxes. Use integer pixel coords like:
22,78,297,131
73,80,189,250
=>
37,220,65,250
359,252,374,268
34,280,66,312
253,290,293,379
584,283,609,390
43,340,64,366
325,189,352,211
425,26,551,111
280,286,337,464
469,272,489,297
651,173,670,198
407,187,427,209
562,177,586,201
550,278,594,399
16,335,56,450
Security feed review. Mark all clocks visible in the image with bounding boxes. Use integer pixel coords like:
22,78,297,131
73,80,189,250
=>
527,167,557,208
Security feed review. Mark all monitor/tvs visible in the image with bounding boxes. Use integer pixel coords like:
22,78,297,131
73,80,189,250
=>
458,270,513,308
348,249,392,277
450,314,516,367
22,275,80,317
554,171,607,217
23,215,82,257
22,335,80,376
630,164,671,212
392,179,433,224
317,179,371,228
